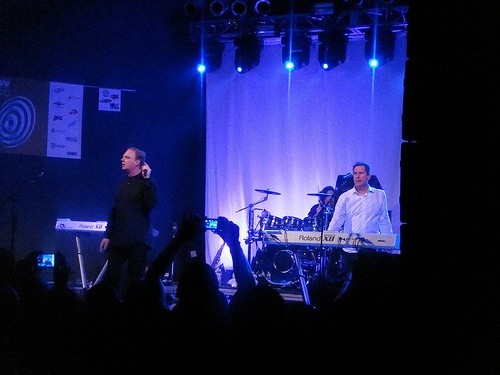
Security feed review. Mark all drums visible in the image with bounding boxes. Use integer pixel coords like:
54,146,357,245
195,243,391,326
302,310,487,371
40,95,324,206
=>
303,217,328,231
260,215,282,230
260,244,316,287
281,216,303,230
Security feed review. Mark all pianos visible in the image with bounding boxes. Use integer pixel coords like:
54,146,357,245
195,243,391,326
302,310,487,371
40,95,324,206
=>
263,230,401,248
55,218,160,237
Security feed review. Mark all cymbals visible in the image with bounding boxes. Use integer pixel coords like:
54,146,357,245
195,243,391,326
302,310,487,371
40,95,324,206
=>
307,194,332,196
255,189,281,195
246,207,264,211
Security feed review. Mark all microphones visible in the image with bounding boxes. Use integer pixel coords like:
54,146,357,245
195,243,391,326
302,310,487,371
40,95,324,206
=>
342,172,351,180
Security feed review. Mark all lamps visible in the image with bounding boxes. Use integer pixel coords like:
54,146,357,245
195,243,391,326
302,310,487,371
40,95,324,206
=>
234,34,261,74
199,37,225,73
364,28,396,70
317,31,349,72
281,31,312,71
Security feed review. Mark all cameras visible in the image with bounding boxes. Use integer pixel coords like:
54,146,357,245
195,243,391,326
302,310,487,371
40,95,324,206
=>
200,218,219,230
35,253,56,267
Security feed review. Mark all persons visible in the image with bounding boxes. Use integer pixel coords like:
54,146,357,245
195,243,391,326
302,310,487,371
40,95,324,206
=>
308,186,335,218
327,162,393,275
99,147,156,287
1,213,402,374
